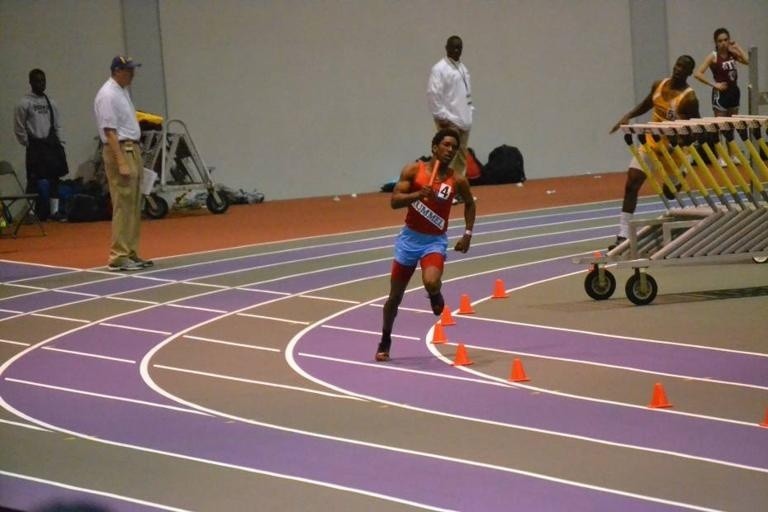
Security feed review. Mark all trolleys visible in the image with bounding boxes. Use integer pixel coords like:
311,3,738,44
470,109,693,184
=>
581,115,765,307
139,119,230,220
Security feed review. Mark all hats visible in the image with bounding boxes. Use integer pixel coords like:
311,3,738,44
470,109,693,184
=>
111,55,141,68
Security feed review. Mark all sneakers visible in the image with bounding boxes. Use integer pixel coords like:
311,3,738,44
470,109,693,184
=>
132,258,153,267
376,339,391,360
108,260,141,270
716,155,742,167
431,293,444,315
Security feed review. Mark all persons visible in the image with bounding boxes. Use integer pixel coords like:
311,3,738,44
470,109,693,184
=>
94,54,154,272
425,35,478,207
13,68,72,224
693,28,750,171
372,129,476,361
607,55,701,252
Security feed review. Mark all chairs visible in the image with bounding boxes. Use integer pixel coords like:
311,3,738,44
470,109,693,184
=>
0,159,47,238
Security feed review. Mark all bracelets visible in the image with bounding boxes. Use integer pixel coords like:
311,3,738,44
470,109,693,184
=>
462,227,473,237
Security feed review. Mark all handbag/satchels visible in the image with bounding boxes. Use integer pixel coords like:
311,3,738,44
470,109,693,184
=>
40,128,68,177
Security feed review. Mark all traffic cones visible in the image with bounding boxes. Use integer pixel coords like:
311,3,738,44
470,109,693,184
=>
506,356,530,381
437,304,457,325
588,251,608,272
429,322,447,345
449,344,474,365
453,294,475,315
644,380,674,409
489,278,509,299
